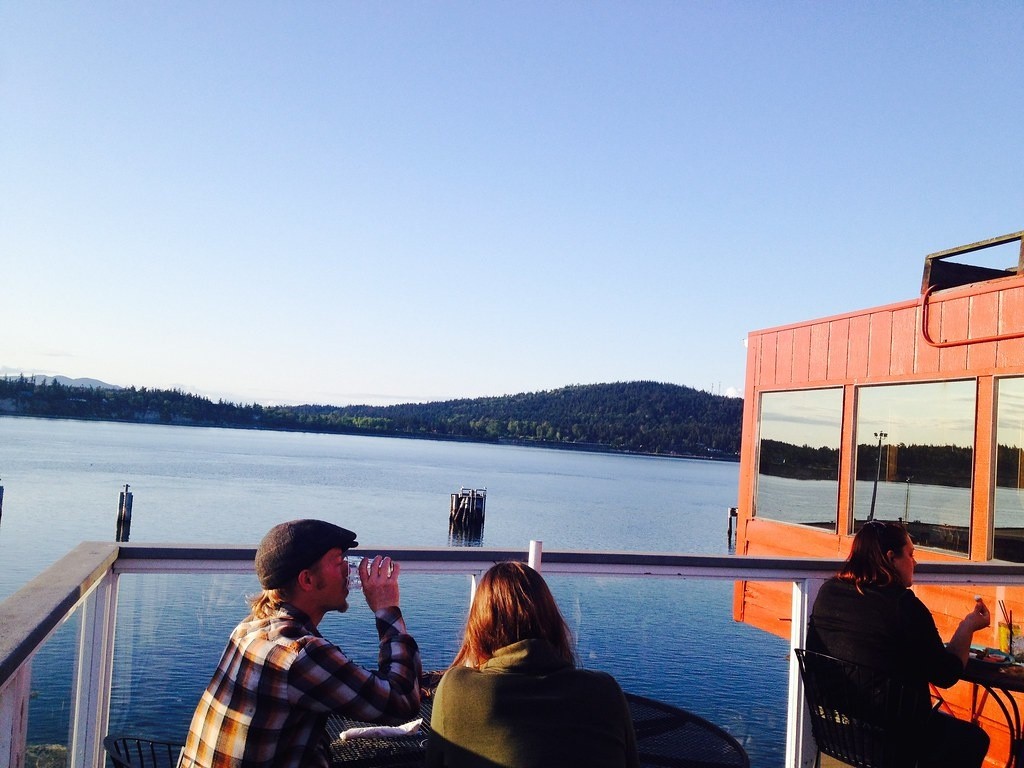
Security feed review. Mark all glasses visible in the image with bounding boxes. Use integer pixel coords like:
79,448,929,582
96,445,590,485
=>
863,521,886,550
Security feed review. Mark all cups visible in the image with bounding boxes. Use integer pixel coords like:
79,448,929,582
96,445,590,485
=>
998,622,1021,653
345,555,394,589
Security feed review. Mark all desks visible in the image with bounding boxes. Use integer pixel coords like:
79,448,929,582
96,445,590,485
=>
929,642,1024,768
295,670,749,768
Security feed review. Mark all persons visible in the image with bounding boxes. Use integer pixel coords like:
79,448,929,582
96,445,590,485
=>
805,521,991,768
431,562,639,768
180,520,421,768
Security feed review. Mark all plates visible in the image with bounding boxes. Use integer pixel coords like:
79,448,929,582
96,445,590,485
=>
944,642,1015,666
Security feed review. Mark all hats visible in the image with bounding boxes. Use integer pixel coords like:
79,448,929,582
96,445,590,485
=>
255,519,359,590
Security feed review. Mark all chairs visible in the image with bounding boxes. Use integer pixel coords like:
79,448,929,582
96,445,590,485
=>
102,733,186,768
796,649,924,768
